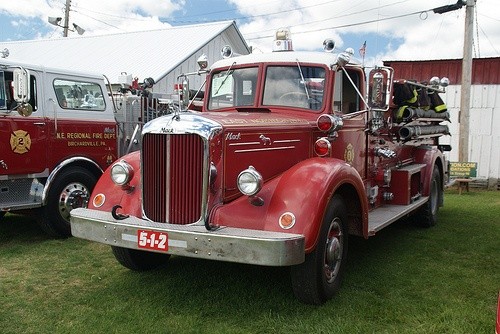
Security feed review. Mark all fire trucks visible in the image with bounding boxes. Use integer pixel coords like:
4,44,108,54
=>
0,46,192,238
68,27,453,308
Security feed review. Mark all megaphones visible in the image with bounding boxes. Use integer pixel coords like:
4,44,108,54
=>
0,47,10,58
72,23,86,36
48,16,62,25
